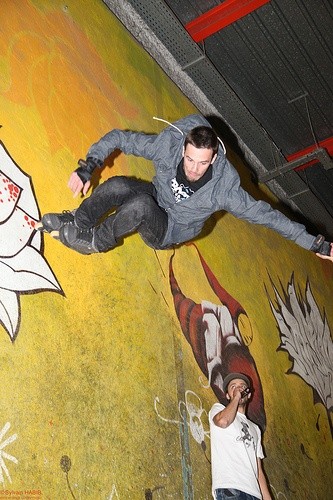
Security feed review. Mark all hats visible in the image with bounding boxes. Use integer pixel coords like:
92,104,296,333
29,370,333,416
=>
223,373,251,394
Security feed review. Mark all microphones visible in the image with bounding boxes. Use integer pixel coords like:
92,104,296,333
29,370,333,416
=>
240,387,251,398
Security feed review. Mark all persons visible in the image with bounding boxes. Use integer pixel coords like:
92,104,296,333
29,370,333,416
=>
208,372,272,500
42,113,333,262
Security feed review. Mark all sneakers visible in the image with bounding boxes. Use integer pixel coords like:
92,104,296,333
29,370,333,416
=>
60,224,98,256
42,210,81,232
